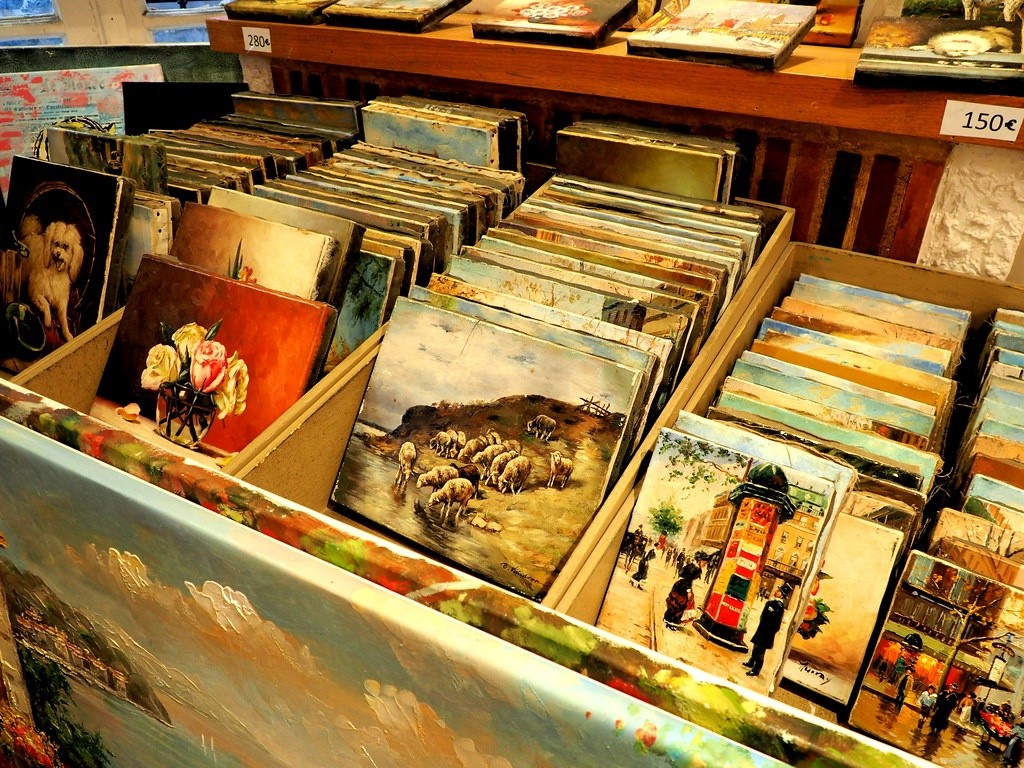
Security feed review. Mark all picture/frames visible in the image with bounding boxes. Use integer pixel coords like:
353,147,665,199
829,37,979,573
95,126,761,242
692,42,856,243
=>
0,0,1024,768
